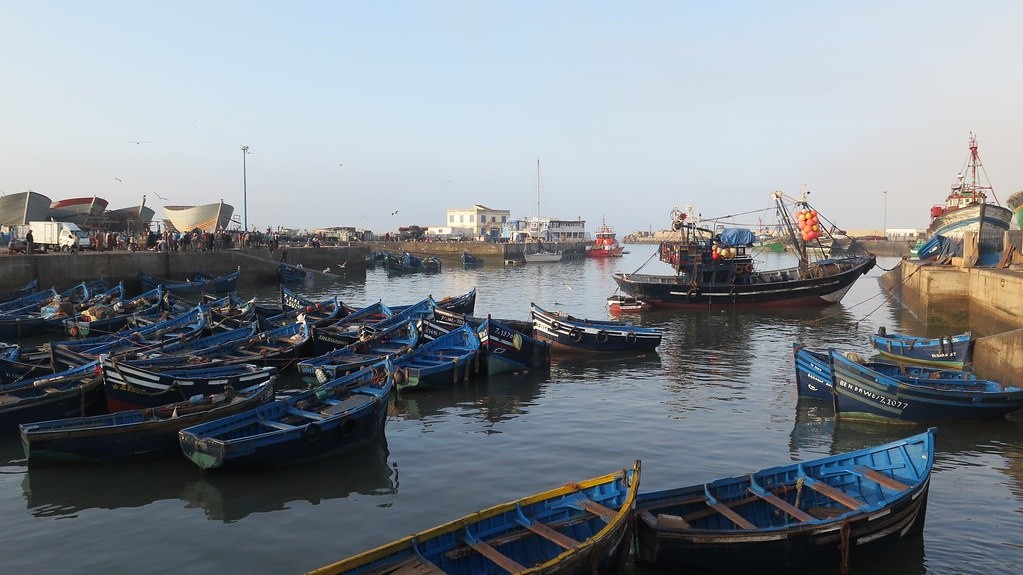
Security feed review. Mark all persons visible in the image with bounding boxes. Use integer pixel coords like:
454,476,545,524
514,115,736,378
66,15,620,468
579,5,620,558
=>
94,228,280,254
280,248,288,263
69,231,80,255
26,230,33,255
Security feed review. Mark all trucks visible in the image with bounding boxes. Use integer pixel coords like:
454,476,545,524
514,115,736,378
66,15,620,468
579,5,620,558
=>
29,221,90,252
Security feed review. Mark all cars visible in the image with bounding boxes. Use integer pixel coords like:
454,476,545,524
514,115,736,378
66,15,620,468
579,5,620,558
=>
8,239,27,253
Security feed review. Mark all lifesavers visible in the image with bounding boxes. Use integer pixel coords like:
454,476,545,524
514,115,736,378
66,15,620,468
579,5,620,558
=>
625,332,637,345
686,287,702,302
568,327,582,343
595,330,609,343
550,321,560,330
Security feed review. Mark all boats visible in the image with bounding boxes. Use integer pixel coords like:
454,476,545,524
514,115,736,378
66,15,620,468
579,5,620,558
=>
793,326,1023,427
301,427,937,575
584,218,625,257
0,189,234,234
365,248,483,271
523,159,563,262
0,266,662,472
612,183,876,309
908,131,1023,266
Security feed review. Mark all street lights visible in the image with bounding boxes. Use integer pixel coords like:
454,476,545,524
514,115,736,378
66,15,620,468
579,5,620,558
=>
241,146,249,231
